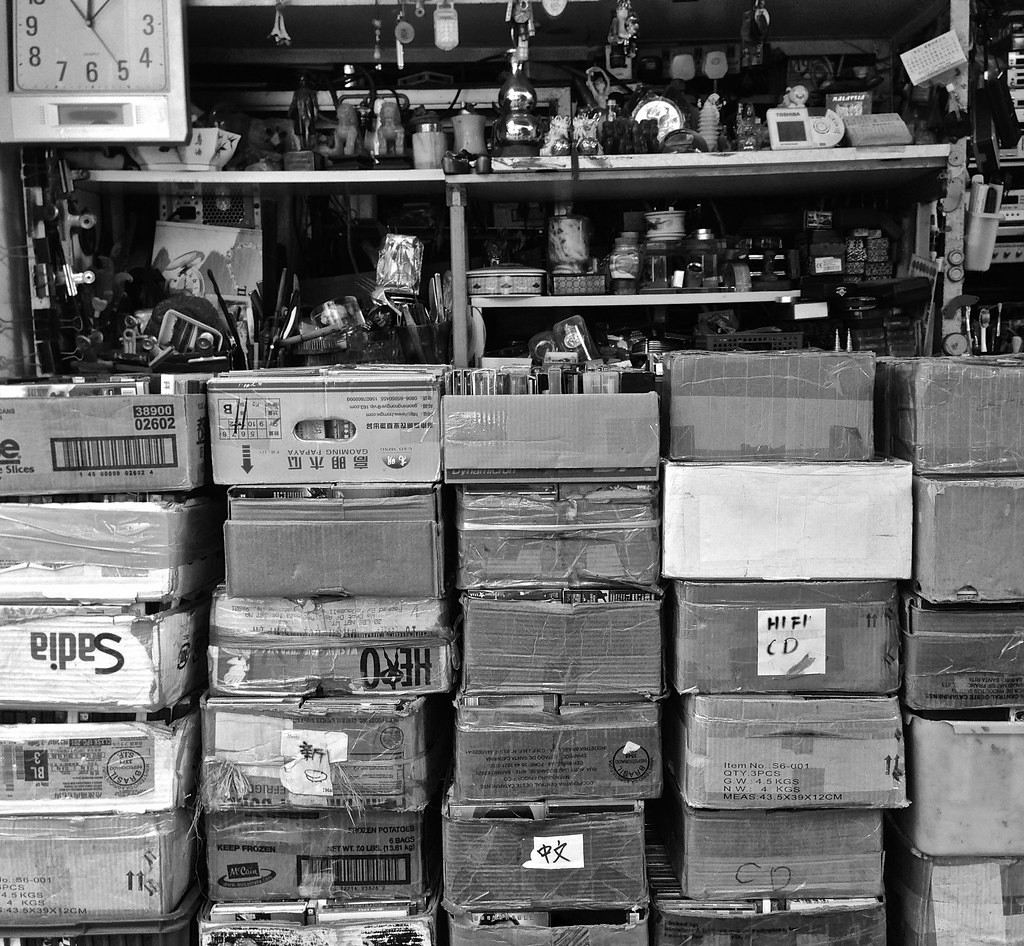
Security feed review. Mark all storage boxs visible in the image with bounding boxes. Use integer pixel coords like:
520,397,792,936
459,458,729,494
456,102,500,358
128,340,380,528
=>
0,352,1024,946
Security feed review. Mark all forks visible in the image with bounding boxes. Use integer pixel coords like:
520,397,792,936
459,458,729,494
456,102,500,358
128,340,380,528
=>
351,273,403,320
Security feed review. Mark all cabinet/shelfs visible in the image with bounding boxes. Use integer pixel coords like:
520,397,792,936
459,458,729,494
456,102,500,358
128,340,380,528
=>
18,0,1024,384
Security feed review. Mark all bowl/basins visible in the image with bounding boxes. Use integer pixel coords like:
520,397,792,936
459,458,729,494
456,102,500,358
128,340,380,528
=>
125,128,242,172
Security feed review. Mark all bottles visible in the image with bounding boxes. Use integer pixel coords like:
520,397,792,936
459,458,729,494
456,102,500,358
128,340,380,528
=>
528,331,560,365
553,314,599,361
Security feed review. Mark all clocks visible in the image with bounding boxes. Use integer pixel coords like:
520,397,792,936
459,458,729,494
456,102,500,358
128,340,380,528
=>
0,0,192,146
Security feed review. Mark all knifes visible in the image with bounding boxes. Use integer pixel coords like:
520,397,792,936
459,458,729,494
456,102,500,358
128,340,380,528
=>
426,277,438,324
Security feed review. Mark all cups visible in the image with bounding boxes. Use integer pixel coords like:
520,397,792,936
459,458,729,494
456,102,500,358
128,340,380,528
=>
963,210,1002,272
450,114,487,155
685,262,704,287
547,215,591,275
411,130,446,169
389,316,450,365
643,210,685,242
